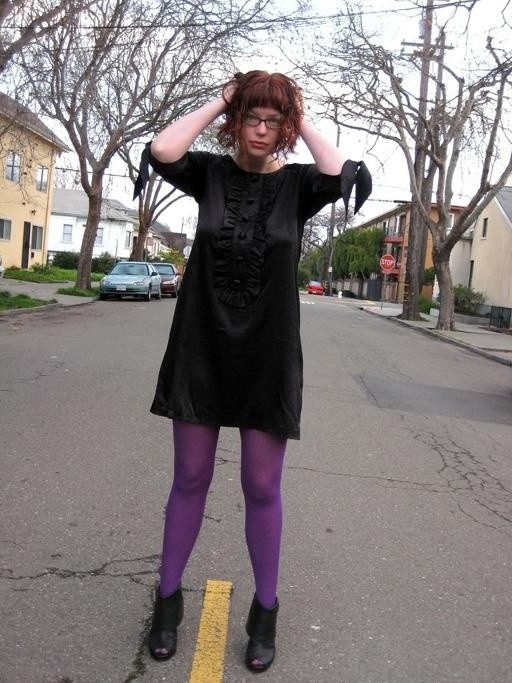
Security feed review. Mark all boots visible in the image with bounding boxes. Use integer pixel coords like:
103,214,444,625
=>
245,592,279,672
148,584,184,662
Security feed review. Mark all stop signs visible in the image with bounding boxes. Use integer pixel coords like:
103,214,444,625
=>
379,254,396,271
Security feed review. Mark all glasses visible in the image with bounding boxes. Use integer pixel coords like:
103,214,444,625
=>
246,114,283,130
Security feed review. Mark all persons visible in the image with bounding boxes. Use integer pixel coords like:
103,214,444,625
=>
145,70,370,673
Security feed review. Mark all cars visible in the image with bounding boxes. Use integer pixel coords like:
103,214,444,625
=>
97,260,163,300
307,279,324,294
150,261,183,298
